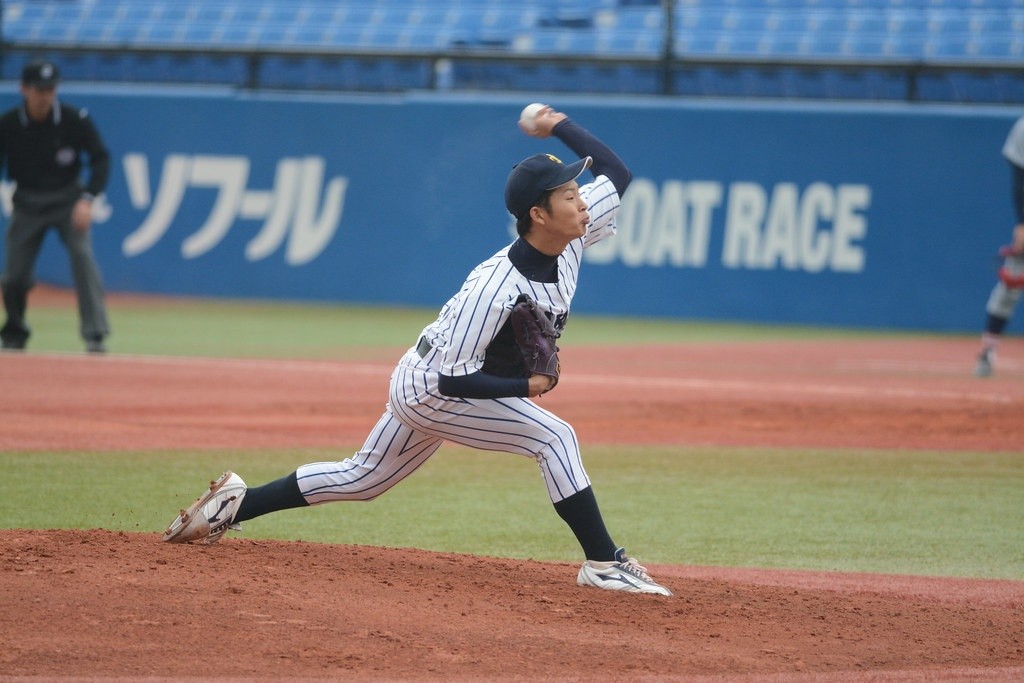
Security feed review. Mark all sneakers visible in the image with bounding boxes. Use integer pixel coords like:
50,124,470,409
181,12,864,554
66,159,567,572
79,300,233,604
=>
163,470,248,544
577,548,673,597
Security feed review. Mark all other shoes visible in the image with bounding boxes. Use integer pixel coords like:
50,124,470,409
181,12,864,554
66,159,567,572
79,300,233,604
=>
86,336,104,352
978,349,996,374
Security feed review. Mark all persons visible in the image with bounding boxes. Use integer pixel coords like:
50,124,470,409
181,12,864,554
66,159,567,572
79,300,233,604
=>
0,58,111,353
162,103,673,595
974,116,1024,378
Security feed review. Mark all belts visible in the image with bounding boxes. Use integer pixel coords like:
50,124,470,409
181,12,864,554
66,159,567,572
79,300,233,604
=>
417,337,432,358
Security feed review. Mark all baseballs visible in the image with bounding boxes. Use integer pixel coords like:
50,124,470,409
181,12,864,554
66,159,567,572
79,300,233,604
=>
520,102,549,132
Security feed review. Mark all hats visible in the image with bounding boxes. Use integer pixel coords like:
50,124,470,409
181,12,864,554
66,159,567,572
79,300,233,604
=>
505,153,593,219
22,61,58,88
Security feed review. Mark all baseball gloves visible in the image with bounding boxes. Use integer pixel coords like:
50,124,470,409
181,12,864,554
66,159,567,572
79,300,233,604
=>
481,293,561,398
